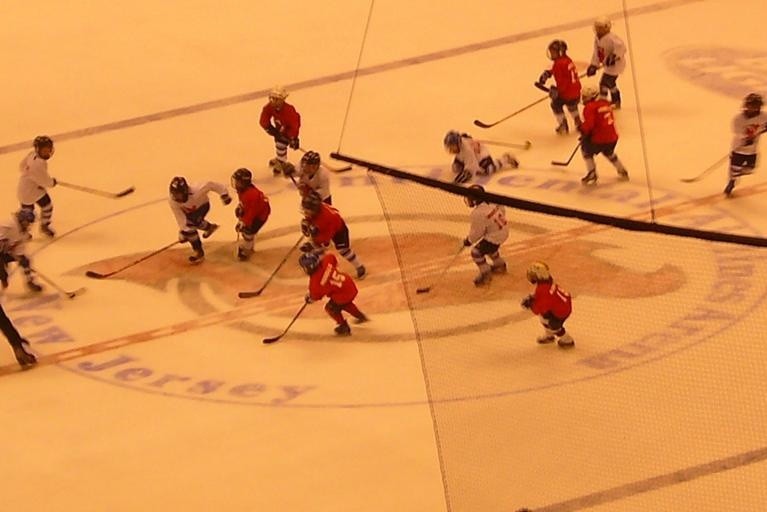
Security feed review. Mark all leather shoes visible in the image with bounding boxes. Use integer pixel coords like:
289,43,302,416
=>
290,138,299,149
463,237,471,246
235,222,244,232
523,296,532,308
236,203,243,217
305,291,313,304
266,124,276,136
221,193,232,204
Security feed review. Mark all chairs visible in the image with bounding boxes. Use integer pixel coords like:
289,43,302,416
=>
42,224,56,237
238,245,254,260
474,263,506,285
189,249,202,261
202,224,217,239
555,120,568,131
356,266,365,279
725,180,735,195
270,159,283,173
334,325,348,334
582,170,596,183
505,152,518,168
575,116,581,125
29,282,42,291
612,91,620,104
538,336,575,346
619,170,628,178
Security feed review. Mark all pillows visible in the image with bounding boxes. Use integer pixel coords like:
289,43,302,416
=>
300,151,321,274
19,210,34,225
268,87,289,110
526,261,550,282
464,184,485,208
34,136,53,159
169,178,189,203
444,133,461,154
547,17,612,100
230,168,252,190
742,94,762,113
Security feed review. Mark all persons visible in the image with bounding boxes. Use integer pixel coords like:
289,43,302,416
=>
17,135,57,237
231,168,271,262
1,302,37,370
167,175,232,263
519,261,575,346
444,129,519,182
575,85,630,182
299,150,333,251
462,184,510,285
259,86,301,174
723,92,766,198
302,191,364,280
1,208,43,292
539,38,583,134
299,251,370,336
587,15,628,108
